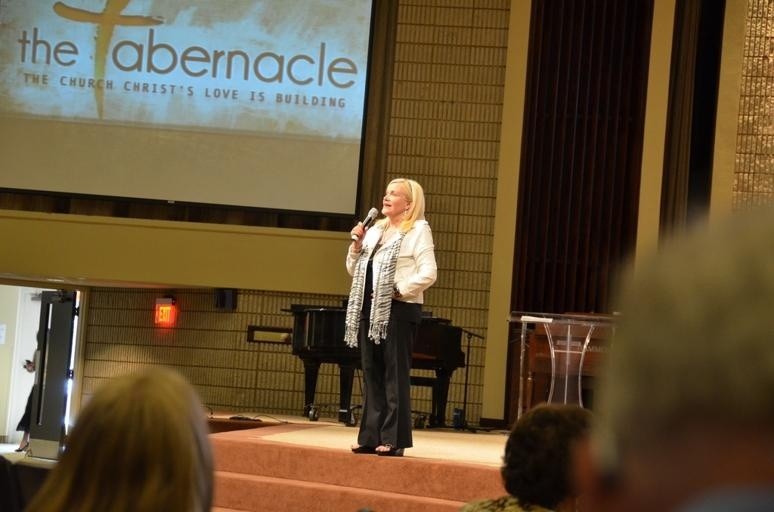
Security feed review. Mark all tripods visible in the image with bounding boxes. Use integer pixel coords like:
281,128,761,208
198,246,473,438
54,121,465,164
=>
426,334,489,433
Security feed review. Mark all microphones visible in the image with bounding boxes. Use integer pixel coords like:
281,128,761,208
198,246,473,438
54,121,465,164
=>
352,208,379,241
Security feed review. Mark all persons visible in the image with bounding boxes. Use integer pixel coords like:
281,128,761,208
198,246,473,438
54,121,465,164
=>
458,403,595,512
346,178,440,457
2,453,51,511
576,195,774,512
22,362,215,512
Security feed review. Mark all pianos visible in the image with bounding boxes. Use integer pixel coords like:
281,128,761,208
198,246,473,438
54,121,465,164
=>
281,299,465,429
506,310,622,428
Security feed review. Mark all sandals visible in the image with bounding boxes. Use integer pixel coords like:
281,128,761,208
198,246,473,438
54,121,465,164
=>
378,444,404,456
352,443,375,452
